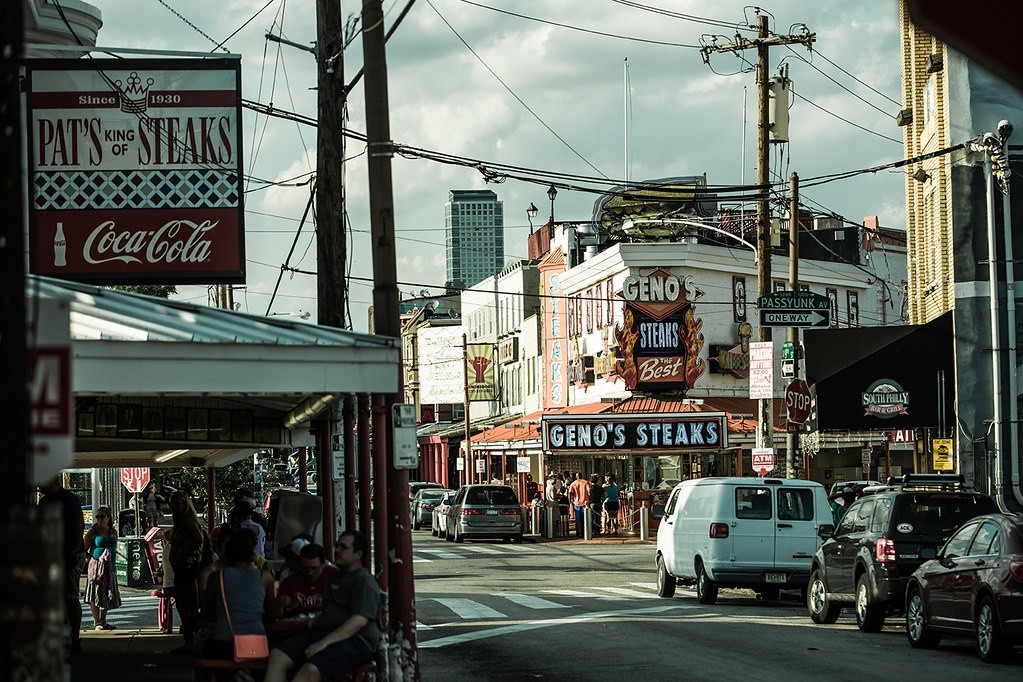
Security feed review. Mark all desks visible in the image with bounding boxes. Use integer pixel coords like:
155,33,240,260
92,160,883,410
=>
192,615,306,630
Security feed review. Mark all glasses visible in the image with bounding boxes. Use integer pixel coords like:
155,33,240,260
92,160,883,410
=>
96,515,107,519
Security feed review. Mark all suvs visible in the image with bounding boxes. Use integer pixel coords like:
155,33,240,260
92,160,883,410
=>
807,472,1001,633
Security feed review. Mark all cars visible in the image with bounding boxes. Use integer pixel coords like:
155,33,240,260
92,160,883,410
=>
254,452,317,491
905,511,1023,665
827,480,884,501
405,479,524,544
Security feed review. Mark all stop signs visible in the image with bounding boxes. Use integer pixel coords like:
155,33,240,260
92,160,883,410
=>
120,466,151,493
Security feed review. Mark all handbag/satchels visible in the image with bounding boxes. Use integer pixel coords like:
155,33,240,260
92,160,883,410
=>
234,633,269,664
95,549,113,589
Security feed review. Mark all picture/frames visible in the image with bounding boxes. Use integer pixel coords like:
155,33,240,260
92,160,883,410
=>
76,402,281,445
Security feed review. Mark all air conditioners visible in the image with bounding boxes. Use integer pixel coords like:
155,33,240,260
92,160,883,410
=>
454,409,464,419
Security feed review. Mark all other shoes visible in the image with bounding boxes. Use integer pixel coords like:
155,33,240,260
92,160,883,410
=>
94,623,104,630
102,623,117,630
171,646,194,656
179,625,184,633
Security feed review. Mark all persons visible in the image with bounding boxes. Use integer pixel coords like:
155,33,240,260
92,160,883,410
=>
826,486,865,530
84,507,123,629
32,448,380,682
490,471,621,537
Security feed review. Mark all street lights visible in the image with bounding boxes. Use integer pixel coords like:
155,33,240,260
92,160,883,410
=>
620,220,776,478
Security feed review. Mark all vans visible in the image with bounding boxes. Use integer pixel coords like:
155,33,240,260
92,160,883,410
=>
654,478,837,608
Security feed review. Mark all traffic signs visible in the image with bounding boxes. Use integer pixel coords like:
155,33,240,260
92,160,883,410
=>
758,308,830,328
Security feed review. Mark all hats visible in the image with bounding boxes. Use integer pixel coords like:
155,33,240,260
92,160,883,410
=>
289,539,310,556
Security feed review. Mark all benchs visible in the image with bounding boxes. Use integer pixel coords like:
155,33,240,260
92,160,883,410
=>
192,656,376,682
